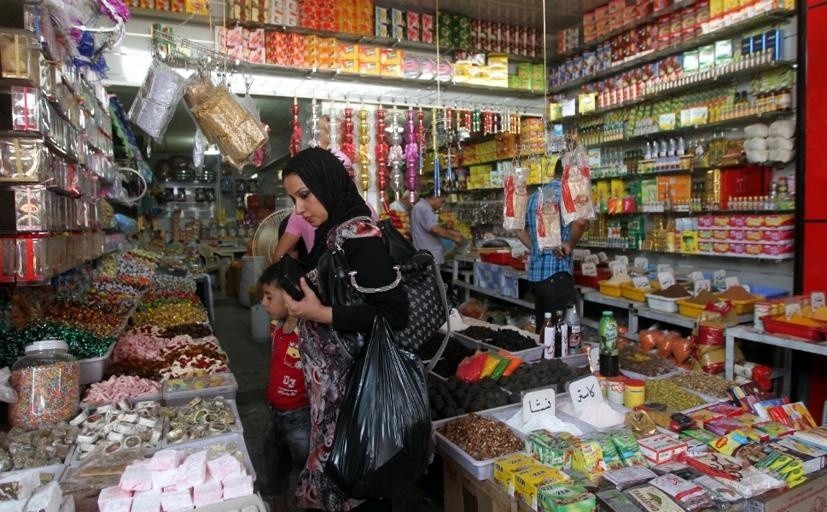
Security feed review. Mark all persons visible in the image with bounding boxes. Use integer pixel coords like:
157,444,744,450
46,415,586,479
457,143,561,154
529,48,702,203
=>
274,149,379,262
261,262,311,511
514,159,588,335
409,188,463,272
389,189,420,213
281,147,409,511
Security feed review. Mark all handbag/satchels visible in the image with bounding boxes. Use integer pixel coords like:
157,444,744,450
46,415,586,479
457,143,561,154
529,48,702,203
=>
379,248,452,353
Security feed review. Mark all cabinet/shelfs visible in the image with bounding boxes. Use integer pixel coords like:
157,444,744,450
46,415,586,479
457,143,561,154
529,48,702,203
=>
0,0,826,512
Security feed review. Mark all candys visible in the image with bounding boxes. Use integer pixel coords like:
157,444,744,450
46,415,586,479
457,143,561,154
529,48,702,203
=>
0,247,254,512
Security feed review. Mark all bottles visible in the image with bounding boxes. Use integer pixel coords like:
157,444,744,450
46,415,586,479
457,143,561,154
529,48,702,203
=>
572,72,791,175
539,303,582,359
7,338,82,430
769,175,788,197
600,310,646,411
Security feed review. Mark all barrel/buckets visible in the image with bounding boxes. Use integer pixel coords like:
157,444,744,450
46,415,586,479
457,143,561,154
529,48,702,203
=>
236,255,267,308
250,303,272,345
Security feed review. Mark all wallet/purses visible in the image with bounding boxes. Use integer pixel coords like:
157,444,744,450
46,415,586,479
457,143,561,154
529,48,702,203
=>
278,254,305,301
